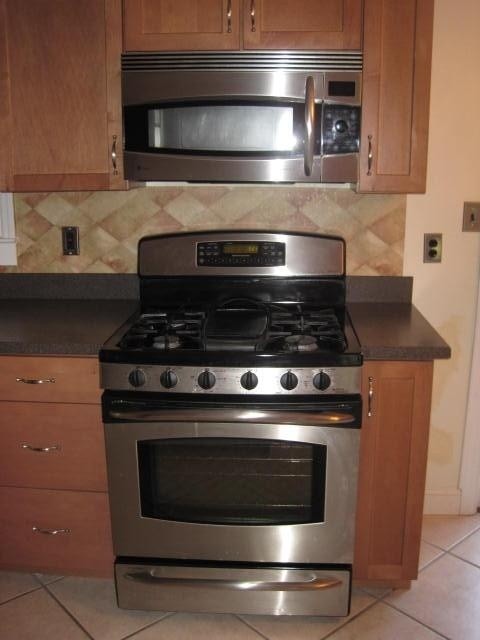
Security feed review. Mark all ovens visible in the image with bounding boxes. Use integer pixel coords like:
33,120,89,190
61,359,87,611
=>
99,400,360,622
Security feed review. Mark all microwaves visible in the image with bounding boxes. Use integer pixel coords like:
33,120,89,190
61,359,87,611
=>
115,48,362,186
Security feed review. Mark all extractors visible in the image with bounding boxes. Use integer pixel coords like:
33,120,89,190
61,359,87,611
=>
115,181,363,193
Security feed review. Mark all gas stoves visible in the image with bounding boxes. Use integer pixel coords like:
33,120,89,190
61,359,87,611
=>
98,281,362,398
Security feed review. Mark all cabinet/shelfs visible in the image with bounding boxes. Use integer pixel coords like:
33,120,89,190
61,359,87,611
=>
354,1,432,193
122,2,364,52
354,357,432,583
2,354,110,578
1,1,124,195
